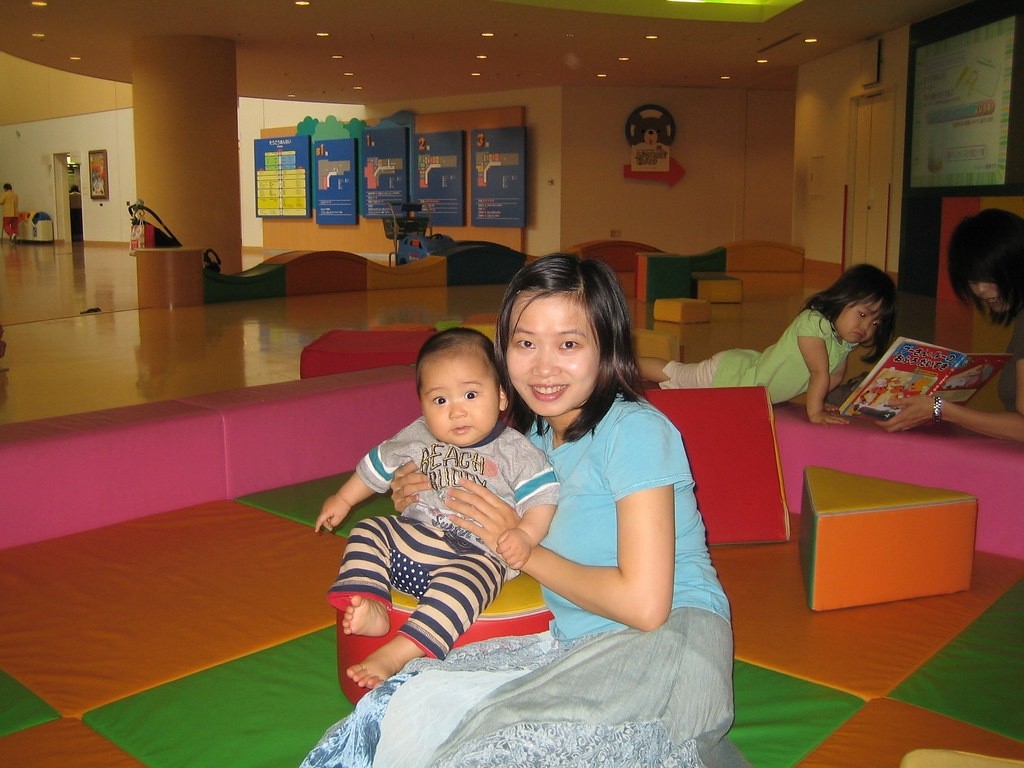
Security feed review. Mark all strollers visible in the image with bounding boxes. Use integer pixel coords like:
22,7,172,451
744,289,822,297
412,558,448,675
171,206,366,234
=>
128,205,222,273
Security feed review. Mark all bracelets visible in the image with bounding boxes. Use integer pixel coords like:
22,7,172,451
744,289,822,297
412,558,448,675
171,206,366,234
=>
932,396,942,423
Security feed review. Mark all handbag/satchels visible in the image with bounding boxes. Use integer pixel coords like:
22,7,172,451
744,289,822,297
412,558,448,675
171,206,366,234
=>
129,215,155,257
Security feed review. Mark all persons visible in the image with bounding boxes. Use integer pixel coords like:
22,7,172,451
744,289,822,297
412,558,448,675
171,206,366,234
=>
315,327,560,689
0,183,19,246
634,264,897,427
875,208,1024,442
69,184,83,243
301,253,733,768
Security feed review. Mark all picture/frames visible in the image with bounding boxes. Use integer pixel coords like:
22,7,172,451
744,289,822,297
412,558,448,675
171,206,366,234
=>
88,149,108,199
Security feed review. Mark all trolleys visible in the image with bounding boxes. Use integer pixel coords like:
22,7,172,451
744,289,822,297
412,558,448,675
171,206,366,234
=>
382,201,453,268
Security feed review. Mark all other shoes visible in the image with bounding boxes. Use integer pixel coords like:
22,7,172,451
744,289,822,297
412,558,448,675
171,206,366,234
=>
11,234,16,243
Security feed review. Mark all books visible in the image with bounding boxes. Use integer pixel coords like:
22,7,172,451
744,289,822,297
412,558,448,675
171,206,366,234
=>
839,336,1013,422
